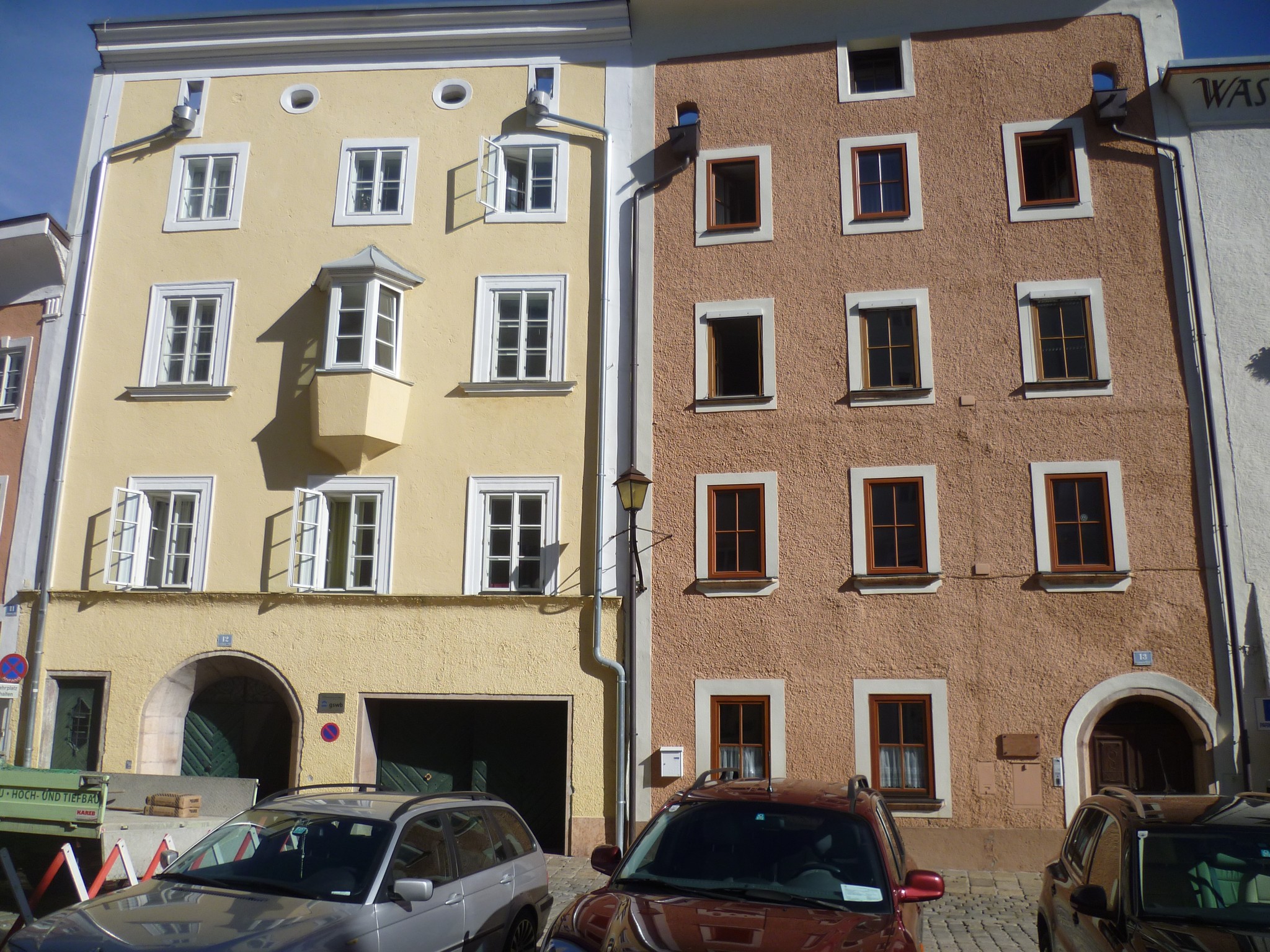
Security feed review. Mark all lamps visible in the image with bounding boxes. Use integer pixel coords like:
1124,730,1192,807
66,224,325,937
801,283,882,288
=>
610,463,673,593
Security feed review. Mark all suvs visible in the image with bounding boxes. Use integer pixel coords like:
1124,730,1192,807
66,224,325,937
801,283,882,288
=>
1036,784,1270,952
4,785,555,949
544,769,950,949
